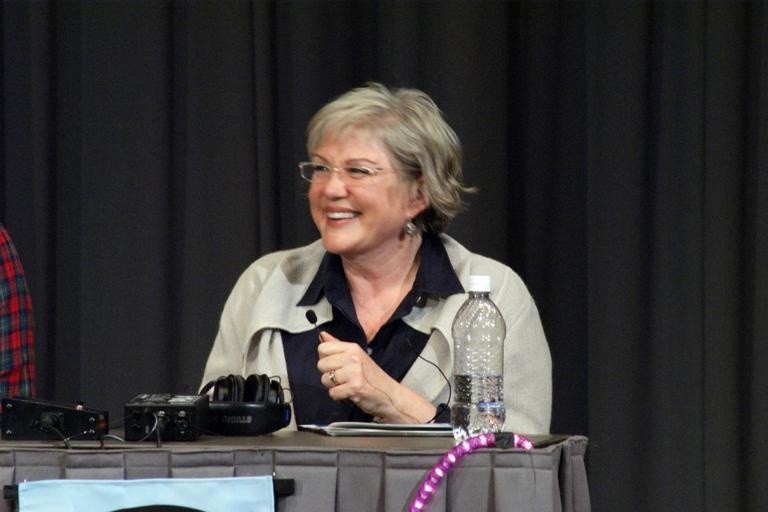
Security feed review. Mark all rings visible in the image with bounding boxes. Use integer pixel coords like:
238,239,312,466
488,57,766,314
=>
327,369,340,386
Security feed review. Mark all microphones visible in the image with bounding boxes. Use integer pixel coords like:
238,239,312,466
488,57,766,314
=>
307,310,452,423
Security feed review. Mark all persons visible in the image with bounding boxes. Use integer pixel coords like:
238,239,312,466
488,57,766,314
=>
193,79,554,439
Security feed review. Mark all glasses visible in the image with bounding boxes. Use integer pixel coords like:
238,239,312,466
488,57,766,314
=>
297,160,413,184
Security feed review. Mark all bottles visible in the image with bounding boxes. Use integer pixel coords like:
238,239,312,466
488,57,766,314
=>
451,275,506,440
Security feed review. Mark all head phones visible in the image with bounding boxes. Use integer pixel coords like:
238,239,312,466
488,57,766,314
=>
197,374,291,436
